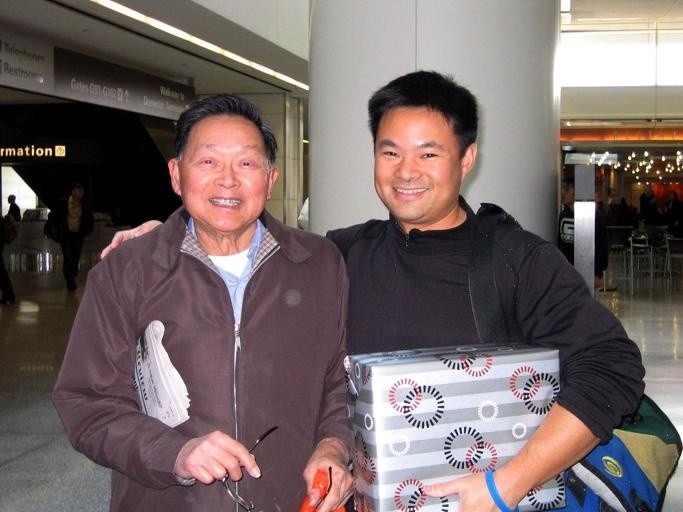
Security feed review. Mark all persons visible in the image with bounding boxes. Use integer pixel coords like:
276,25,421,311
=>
1,215,17,305
7,196,20,222
560,176,610,289
100,69,647,511
43,181,95,291
52,91,357,511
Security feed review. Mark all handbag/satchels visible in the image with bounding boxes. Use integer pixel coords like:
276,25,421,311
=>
549,393,682,512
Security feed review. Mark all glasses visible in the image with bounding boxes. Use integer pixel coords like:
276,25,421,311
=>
222,427,332,512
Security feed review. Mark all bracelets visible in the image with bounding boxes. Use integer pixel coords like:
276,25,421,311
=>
483,468,519,512
